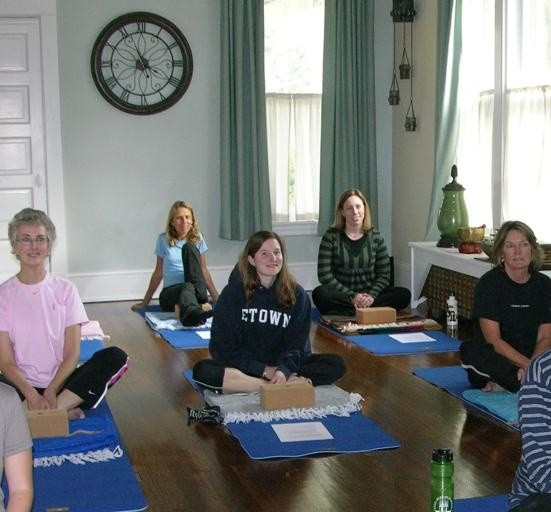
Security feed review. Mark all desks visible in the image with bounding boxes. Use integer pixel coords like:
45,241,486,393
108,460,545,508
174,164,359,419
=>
406,240,551,310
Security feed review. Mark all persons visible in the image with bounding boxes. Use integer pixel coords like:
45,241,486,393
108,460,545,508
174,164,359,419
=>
310,188,410,314
457,221,551,393
191,232,347,394
0,207,129,419
0,383,36,512
508,347,550,512
132,200,221,327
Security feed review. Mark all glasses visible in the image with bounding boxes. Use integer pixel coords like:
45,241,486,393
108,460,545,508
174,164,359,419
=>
17,235,48,245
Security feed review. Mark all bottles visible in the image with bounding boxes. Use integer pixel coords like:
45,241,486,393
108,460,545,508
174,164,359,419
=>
430,448,455,512
447,292,459,326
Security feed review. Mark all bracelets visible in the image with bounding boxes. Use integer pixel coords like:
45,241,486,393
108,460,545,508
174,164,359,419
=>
262,367,267,378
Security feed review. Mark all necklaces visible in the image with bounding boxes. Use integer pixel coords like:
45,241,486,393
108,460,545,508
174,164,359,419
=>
345,230,362,237
174,243,183,249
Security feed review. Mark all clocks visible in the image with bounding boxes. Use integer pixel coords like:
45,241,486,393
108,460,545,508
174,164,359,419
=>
91,11,195,117
